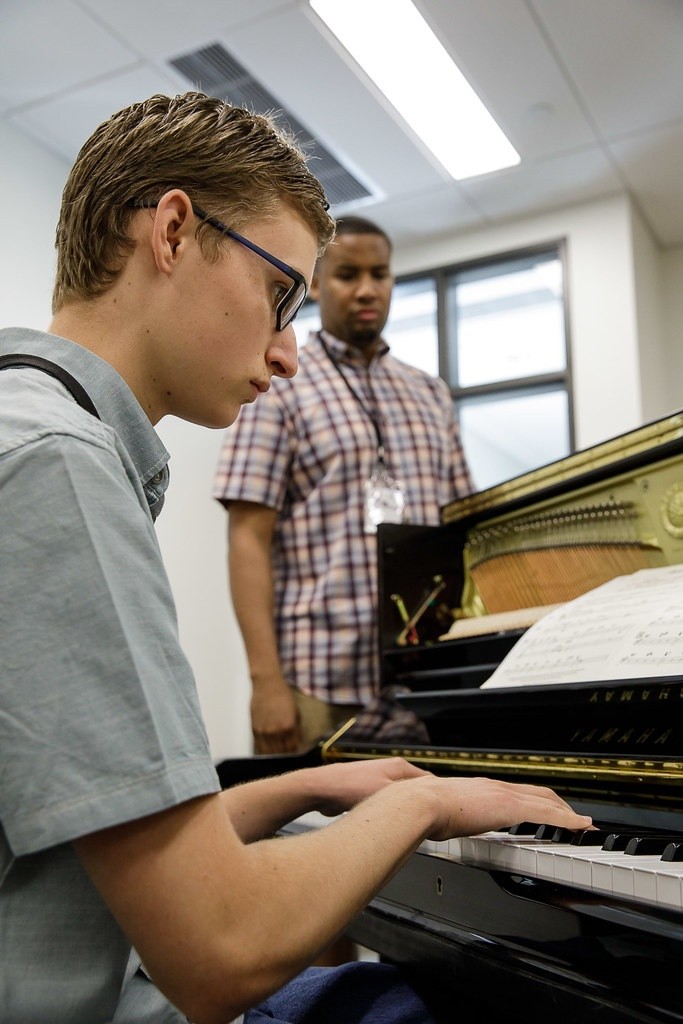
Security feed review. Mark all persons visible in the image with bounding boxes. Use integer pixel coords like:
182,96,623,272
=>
213,216,478,967
0,90,600,1024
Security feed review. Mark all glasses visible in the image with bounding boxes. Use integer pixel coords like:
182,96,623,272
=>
136,195,310,333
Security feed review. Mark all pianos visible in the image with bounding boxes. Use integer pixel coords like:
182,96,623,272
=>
213,406,683,1024
